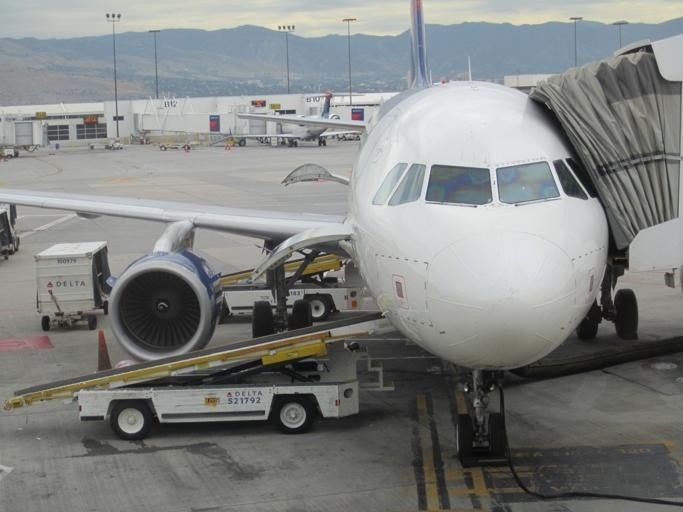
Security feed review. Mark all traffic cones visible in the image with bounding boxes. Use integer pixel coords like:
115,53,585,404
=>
95,330,111,373
